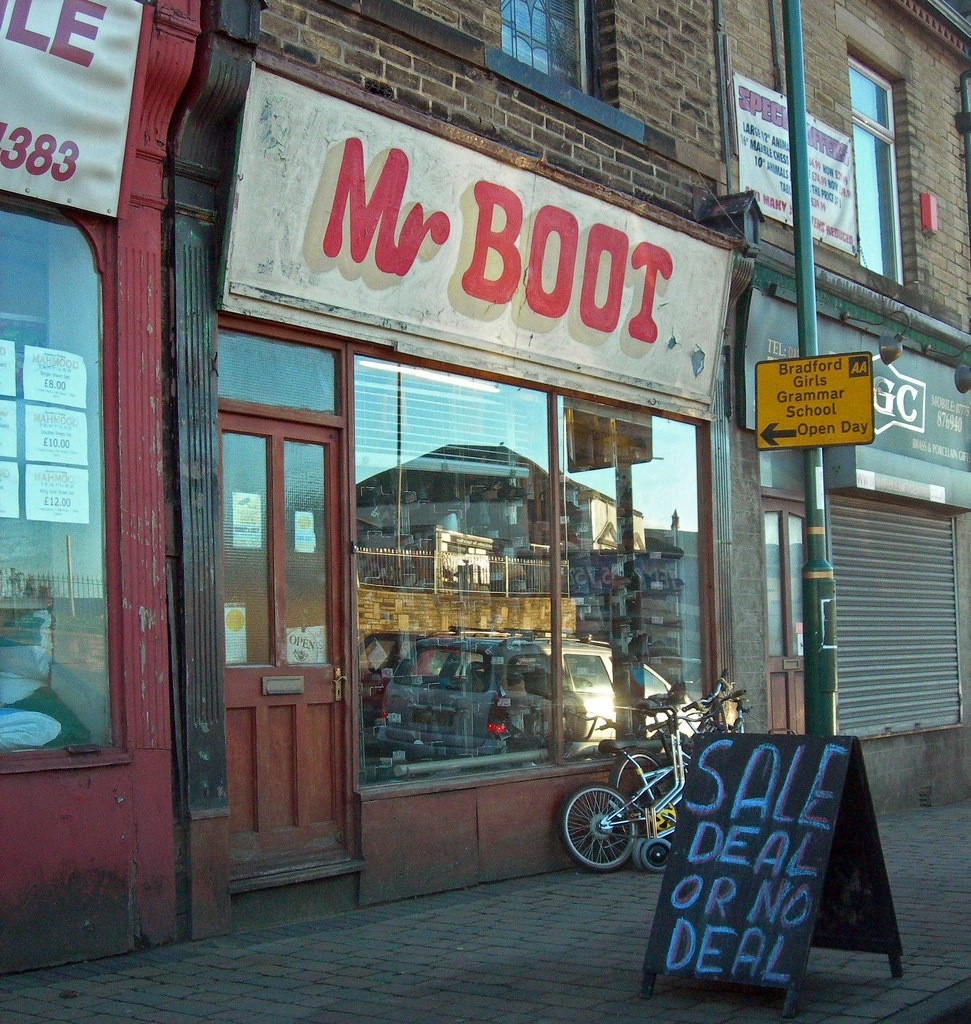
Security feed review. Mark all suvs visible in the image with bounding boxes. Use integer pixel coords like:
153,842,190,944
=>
357,627,543,749
375,631,706,769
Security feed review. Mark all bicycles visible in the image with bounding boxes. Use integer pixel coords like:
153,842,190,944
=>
557,667,756,876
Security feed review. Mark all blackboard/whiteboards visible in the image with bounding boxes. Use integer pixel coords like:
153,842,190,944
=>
641,733,855,992
